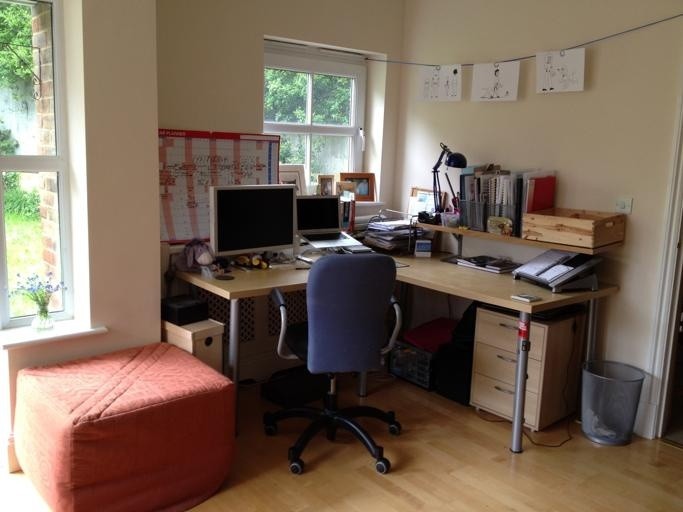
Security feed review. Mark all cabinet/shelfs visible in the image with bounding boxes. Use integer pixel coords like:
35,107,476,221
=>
468,306,587,433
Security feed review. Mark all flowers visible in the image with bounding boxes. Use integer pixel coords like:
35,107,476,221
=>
4,271,68,316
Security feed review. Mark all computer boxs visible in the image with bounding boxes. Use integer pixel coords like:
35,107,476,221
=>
388,317,459,392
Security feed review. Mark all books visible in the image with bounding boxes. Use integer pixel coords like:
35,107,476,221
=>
456,164,603,288
338,190,355,231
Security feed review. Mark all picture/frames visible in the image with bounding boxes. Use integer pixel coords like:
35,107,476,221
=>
408,188,447,218
339,172,375,202
317,175,335,197
279,163,307,195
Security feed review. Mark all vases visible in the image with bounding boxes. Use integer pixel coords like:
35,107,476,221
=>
31,306,54,329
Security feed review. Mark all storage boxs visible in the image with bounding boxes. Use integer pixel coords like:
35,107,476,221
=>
521,206,626,248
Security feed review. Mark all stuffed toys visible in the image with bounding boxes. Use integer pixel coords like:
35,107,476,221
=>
164,238,216,284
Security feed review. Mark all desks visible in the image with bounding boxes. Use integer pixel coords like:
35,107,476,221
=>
172,253,619,454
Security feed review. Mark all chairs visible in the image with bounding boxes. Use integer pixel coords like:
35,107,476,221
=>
263,251,403,475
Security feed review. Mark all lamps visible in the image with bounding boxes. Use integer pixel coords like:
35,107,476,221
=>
431,142,468,225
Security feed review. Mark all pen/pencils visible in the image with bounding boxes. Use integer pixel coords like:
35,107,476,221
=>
456,190,459,208
445,172,456,201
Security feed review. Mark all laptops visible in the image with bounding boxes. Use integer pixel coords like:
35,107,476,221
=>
296,195,363,249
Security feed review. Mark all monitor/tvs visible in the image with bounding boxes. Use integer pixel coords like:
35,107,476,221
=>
209,184,298,256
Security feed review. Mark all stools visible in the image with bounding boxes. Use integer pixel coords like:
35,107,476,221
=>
13,342,238,512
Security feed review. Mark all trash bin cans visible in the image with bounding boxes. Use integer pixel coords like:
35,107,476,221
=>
579,358,646,449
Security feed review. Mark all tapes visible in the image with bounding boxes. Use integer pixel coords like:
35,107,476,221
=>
424,212,435,218
